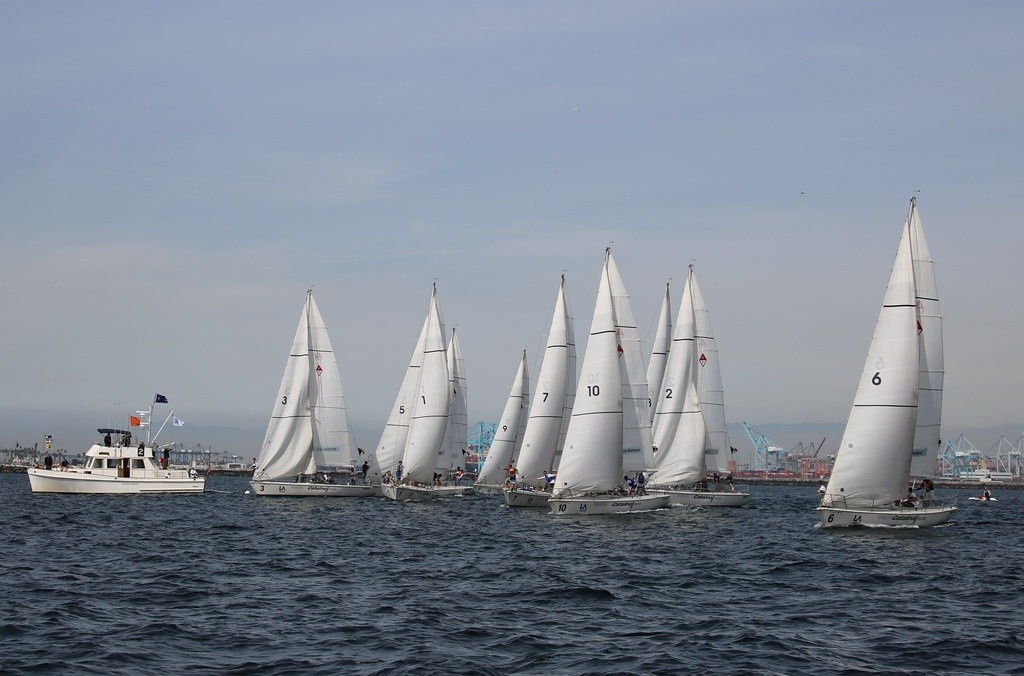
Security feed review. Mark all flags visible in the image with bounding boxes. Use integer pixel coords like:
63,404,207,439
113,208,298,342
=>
130,416,140,426
156,394,168,403
173,417,184,426
44,435,54,448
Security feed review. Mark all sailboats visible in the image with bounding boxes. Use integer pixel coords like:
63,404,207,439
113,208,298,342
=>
503,273,577,508
625,263,749,507
364,281,450,501
813,196,957,529
549,246,672,515
246,290,376,496
433,326,475,495
473,348,530,497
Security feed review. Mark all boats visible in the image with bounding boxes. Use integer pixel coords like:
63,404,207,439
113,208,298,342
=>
968,496,999,501
25,401,210,493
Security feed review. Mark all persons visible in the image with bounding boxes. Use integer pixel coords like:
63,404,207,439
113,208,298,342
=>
713,473,719,484
983,487,991,500
498,464,519,489
537,471,555,493
455,467,463,486
104,433,111,446
909,487,916,497
818,483,826,493
636,473,649,495
382,461,404,484
45,454,52,470
362,461,370,481
914,480,936,499
60,460,68,471
163,448,172,469
623,473,637,496
250,458,256,481
